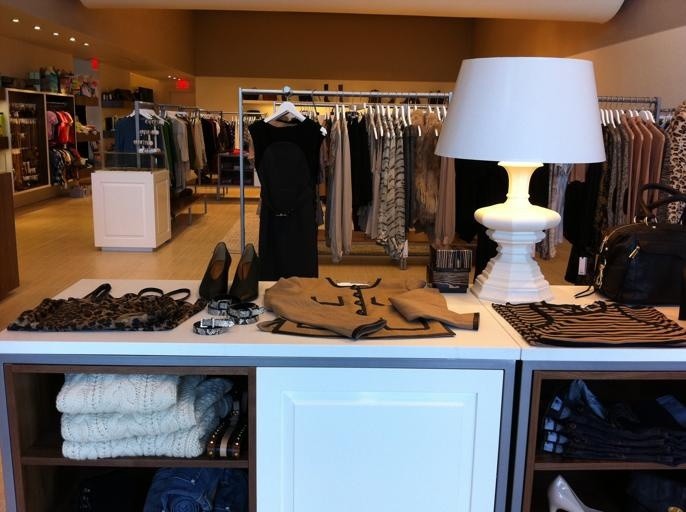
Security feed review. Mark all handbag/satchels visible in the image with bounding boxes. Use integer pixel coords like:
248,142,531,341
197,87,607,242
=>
596,185,683,308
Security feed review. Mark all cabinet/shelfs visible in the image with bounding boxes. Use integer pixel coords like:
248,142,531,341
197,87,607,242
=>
5,88,80,196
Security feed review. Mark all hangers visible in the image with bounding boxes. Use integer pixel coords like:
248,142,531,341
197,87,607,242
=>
248,92,448,140
125,104,164,124
591,95,655,128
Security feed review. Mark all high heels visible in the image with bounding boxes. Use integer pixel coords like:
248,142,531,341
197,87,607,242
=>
544,474,602,512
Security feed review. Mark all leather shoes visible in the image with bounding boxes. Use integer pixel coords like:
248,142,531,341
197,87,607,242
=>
198,240,233,302
229,243,259,300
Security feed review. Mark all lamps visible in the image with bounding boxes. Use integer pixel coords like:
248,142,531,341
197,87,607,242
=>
166,71,190,90
433,54,606,304
